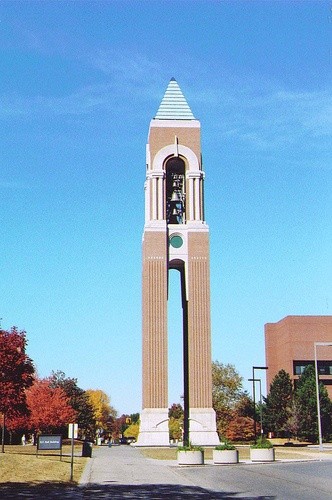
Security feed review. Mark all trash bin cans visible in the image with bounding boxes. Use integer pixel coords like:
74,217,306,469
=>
82,440,93,457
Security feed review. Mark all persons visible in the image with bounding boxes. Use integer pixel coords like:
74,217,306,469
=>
22,434,26,446
